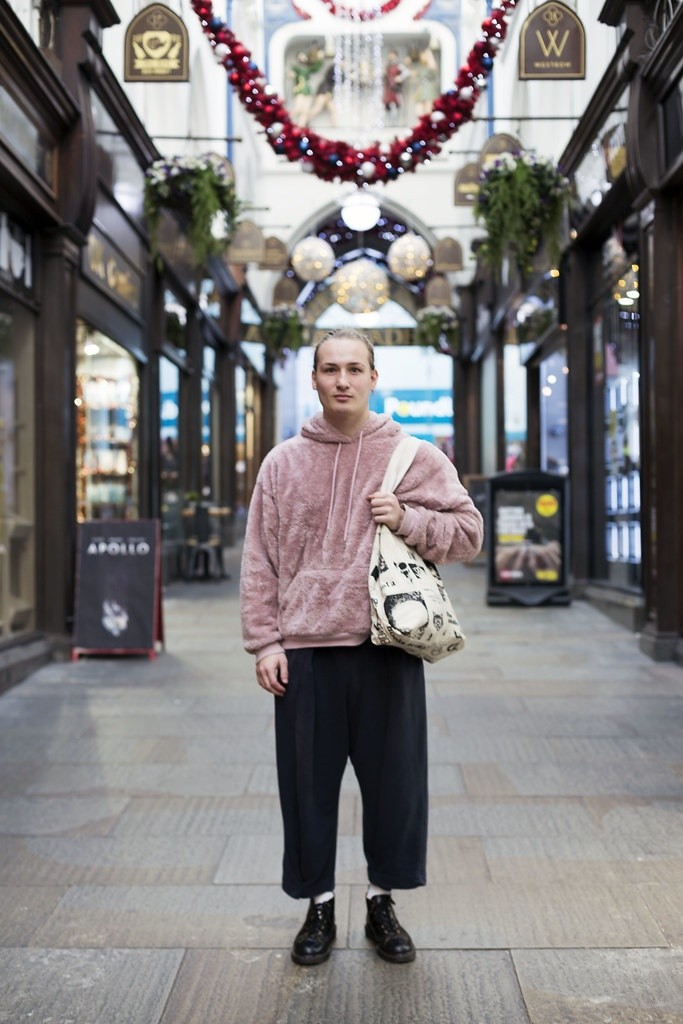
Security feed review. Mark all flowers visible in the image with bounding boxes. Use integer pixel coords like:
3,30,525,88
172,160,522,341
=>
261,303,312,367
516,291,558,339
415,306,463,356
142,150,244,274
469,148,578,289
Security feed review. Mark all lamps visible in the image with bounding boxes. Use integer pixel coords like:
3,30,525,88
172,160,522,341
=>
292,0,435,314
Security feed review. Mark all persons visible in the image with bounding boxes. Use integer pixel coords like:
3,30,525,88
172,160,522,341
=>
241,330,483,966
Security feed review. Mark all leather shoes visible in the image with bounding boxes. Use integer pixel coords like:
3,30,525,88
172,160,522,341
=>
291,892,337,965
365,892,416,962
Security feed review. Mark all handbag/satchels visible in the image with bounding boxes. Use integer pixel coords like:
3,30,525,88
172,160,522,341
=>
368,524,466,664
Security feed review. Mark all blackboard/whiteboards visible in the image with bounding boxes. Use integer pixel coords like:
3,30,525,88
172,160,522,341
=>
72,518,164,651
